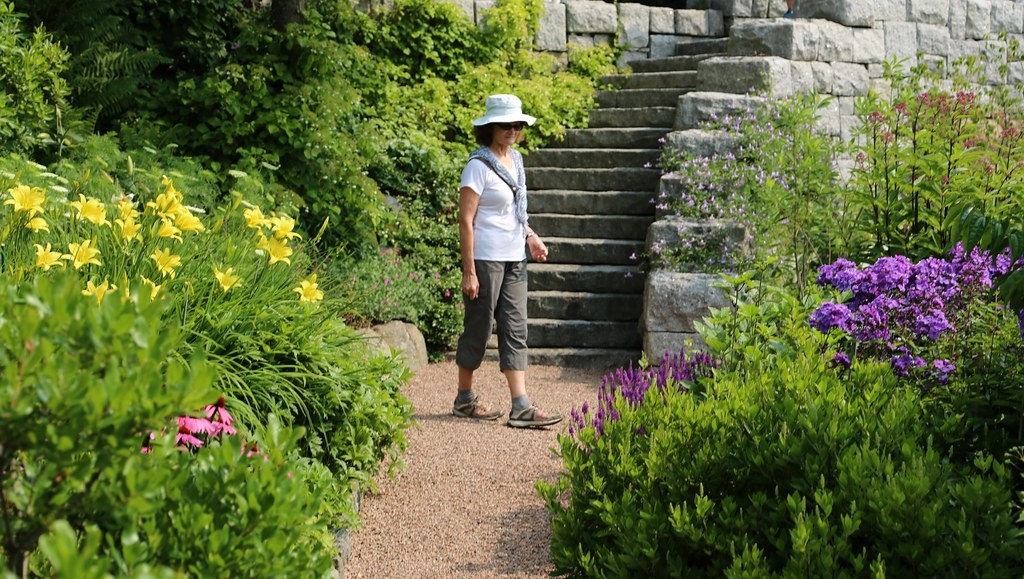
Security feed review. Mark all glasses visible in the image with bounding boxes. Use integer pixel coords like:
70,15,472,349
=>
495,122,523,131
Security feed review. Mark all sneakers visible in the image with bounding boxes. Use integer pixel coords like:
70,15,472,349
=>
507,401,563,427
452,395,504,421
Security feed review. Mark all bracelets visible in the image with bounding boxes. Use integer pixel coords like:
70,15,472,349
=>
525,230,534,239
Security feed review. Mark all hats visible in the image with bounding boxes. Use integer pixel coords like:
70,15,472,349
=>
471,94,537,126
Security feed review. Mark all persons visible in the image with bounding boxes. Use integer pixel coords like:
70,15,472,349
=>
448,93,566,430
